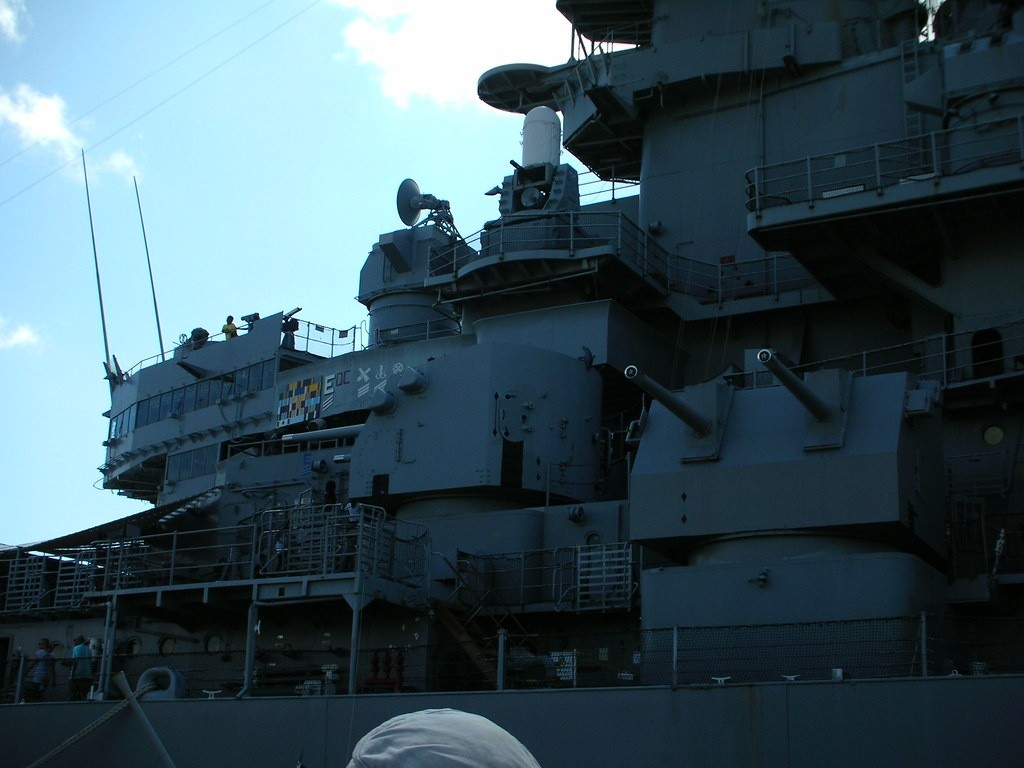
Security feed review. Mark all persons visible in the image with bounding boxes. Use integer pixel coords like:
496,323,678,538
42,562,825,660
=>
221,316,237,341
67,634,98,701
23,638,57,703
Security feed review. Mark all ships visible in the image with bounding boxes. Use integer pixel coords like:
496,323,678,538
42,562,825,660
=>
0,0,1024,768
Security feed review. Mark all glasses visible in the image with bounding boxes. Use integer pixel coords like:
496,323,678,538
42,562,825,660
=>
74,639,79,641
39,643,41,646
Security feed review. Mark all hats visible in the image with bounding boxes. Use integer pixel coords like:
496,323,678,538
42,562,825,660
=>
347,708,542,768
